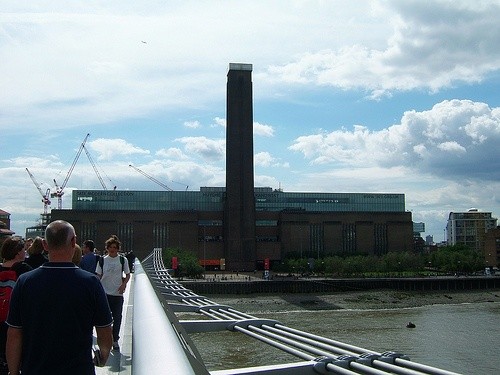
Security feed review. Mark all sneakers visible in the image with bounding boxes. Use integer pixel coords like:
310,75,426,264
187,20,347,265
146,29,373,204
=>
113,341,119,350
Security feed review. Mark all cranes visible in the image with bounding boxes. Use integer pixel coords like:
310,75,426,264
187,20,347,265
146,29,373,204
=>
25,167,51,225
51,132,90,209
128,164,189,191
81,142,117,190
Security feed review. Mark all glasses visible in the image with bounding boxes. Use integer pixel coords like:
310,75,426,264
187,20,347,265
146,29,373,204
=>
15,239,24,248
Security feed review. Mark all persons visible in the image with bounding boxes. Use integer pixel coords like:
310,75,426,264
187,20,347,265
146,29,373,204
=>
0,236,34,375
95,235,130,351
5,220,115,375
0,235,135,275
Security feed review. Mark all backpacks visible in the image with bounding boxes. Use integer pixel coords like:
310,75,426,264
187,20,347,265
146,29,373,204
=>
0,261,25,323
